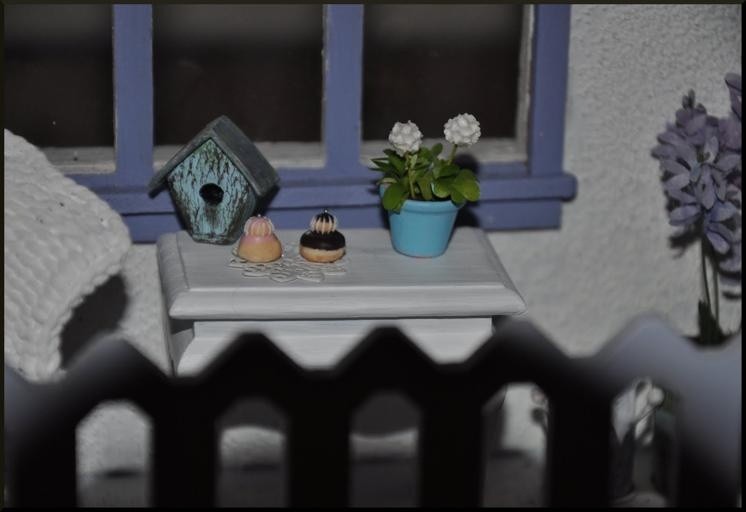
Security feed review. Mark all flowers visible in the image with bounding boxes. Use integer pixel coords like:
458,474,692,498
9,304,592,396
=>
652,74,742,346
372,113,480,214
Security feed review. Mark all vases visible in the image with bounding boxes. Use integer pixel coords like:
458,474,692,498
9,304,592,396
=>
382,186,465,257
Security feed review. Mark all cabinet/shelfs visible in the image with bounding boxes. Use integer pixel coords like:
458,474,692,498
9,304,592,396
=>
159,226,527,379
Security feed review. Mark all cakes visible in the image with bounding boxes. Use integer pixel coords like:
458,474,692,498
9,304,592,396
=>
300,213,345,263
237,215,281,263
